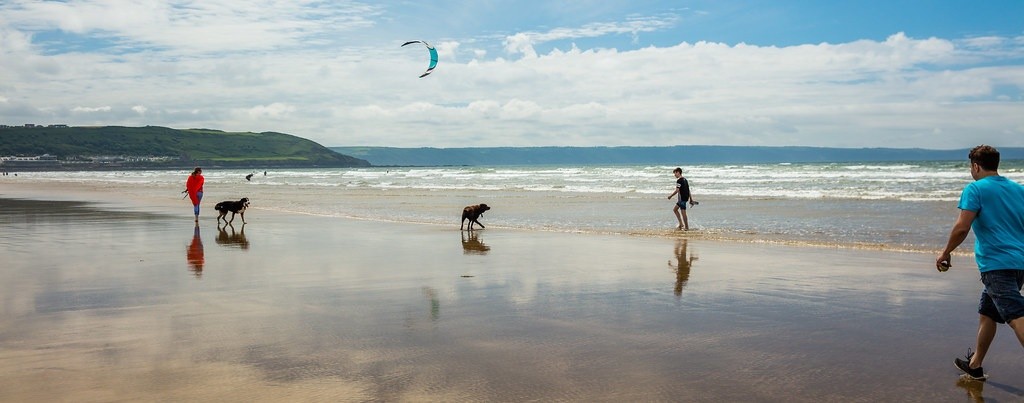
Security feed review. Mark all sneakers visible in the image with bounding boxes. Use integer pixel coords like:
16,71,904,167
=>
953,348,984,379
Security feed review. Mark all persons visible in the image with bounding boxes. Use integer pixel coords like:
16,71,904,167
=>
186,167,204,222
668,168,693,231
246,173,253,181
936,145,1024,379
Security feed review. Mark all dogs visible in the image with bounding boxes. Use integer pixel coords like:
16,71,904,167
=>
461,203,490,230
215,197,250,224
